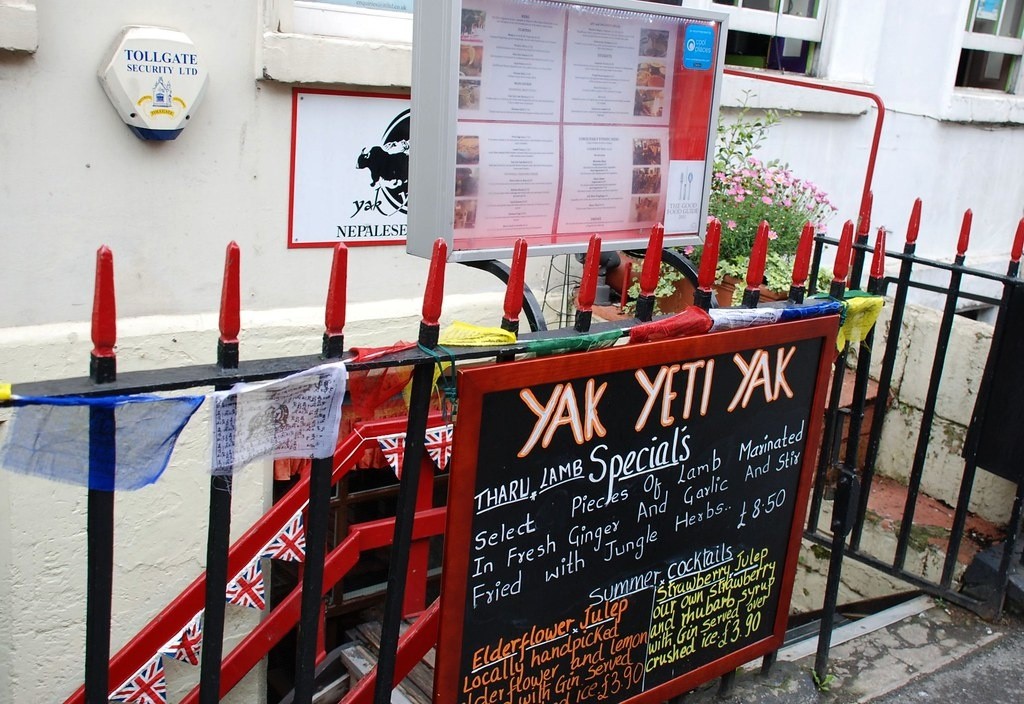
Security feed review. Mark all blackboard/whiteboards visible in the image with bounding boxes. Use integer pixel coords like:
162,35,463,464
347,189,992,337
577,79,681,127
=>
433,315,843,704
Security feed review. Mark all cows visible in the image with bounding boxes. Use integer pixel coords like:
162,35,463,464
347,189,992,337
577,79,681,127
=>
355,145,409,187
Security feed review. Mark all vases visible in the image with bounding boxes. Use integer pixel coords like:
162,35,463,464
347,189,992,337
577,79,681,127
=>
605,250,643,298
658,265,789,315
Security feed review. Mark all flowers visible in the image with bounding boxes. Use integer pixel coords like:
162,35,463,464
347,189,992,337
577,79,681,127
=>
606,86,839,315
